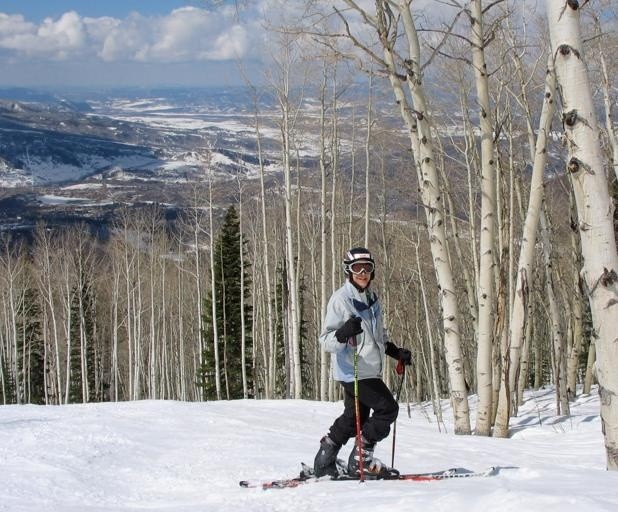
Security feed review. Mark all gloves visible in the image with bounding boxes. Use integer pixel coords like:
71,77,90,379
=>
335,315,363,344
386,343,412,365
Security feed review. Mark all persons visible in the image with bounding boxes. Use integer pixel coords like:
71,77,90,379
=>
312,245,413,480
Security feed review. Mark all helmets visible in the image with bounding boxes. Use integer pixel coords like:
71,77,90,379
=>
343,246,375,280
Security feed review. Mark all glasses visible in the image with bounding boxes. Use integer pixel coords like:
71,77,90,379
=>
345,261,374,274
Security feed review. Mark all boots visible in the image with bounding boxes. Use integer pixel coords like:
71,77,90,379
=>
314,437,347,474
348,439,386,473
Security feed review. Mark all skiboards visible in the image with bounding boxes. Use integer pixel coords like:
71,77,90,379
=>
239,467,498,490
